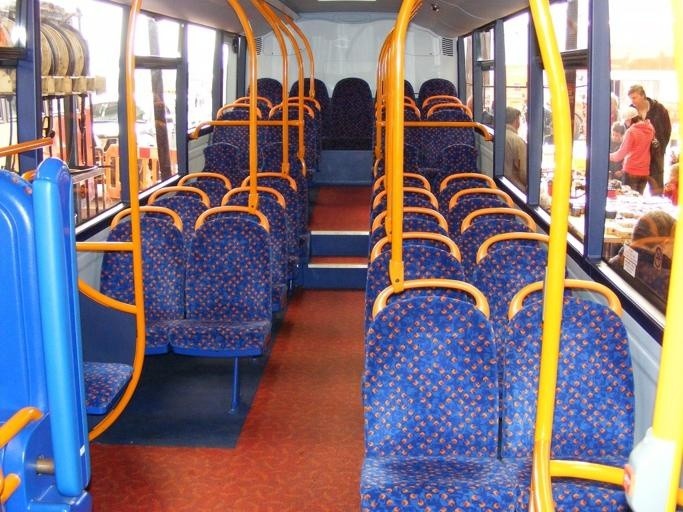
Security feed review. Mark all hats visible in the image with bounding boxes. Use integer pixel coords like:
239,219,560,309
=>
619,107,638,126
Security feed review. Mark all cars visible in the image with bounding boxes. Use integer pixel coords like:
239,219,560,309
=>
499,71,586,143
85,99,172,145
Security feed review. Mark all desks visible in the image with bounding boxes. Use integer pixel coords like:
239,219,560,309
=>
539,169,678,260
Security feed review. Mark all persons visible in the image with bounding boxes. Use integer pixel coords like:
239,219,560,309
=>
608,126,625,180
624,85,671,197
504,108,527,193
664,164,679,206
609,106,655,195
